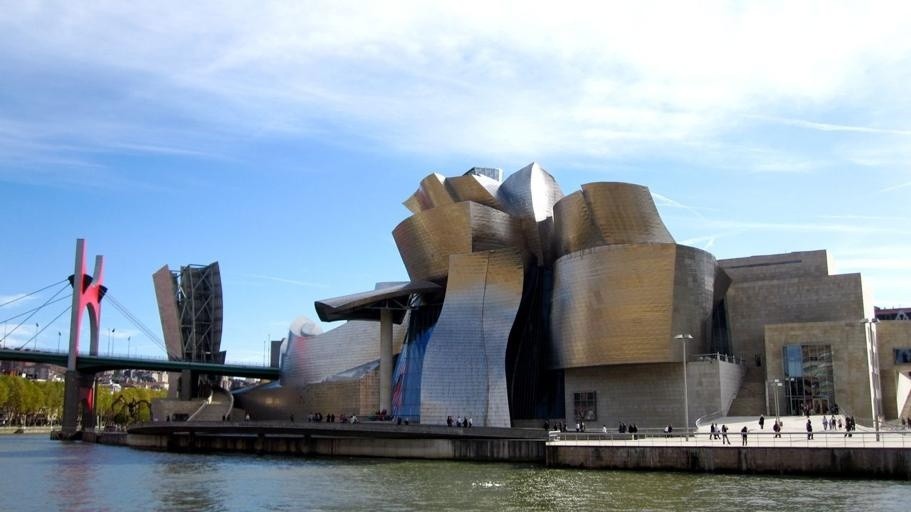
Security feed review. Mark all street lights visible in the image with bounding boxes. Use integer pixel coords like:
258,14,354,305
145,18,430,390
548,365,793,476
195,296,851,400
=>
860,316,880,439
676,334,693,442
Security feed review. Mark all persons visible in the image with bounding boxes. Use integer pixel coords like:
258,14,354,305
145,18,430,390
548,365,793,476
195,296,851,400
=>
772,422,782,438
721,425,731,445
807,421,813,439
544,421,673,440
306,411,360,424
245,413,250,426
227,414,232,425
221,413,226,425
741,425,748,446
823,414,856,437
759,414,765,429
714,423,722,439
806,418,813,438
165,413,171,424
709,422,718,440
287,413,298,427
446,415,473,428
